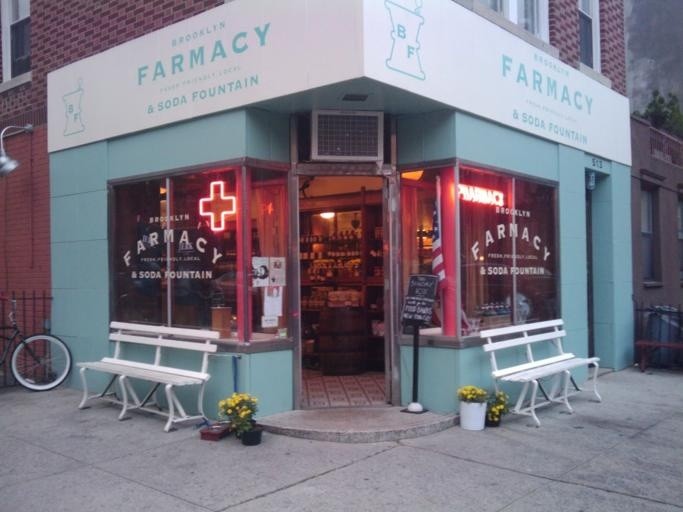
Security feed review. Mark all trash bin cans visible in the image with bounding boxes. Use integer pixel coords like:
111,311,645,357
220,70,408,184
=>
648,306,678,369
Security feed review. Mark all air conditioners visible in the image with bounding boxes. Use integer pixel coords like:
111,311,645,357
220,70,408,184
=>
309,106,385,164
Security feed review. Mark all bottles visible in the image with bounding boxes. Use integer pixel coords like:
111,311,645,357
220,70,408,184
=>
299,230,383,307
478,300,511,316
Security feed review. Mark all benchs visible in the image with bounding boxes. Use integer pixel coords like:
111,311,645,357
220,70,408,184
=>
75,320,221,433
634,338,683,373
478,317,603,429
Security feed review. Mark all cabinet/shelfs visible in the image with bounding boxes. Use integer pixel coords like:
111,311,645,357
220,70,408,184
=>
300,202,385,313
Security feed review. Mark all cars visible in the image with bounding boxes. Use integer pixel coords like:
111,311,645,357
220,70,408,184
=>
197,260,259,308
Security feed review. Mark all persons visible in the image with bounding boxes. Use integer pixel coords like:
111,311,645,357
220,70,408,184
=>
255,265,268,280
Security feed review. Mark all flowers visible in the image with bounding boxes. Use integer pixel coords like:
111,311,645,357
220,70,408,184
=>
217,391,259,438
457,385,487,403
486,388,511,423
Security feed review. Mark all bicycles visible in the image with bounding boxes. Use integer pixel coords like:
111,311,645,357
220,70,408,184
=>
0,296,72,391
112,270,161,324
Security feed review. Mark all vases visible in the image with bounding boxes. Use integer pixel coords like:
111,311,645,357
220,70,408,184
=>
458,400,488,431
483,410,501,428
241,424,263,446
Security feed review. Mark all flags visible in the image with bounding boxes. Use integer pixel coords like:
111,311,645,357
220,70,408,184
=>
428,176,448,328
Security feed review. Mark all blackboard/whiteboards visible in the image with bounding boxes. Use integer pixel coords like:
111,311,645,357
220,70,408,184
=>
401,275,437,329
